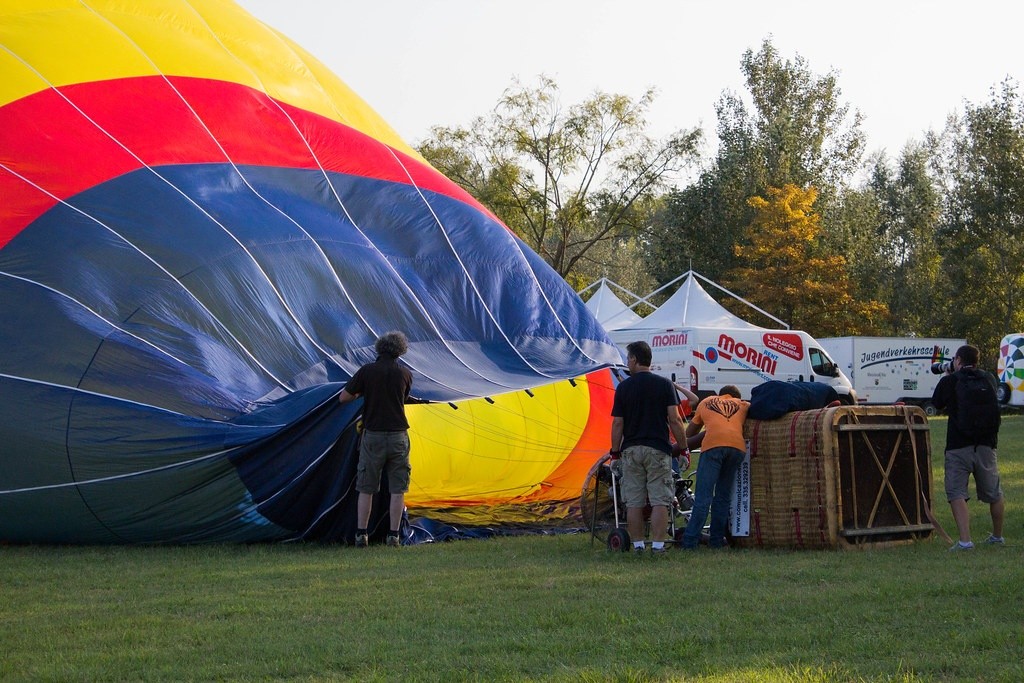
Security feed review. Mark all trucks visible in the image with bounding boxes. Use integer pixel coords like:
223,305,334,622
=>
646,327,859,418
814,333,965,417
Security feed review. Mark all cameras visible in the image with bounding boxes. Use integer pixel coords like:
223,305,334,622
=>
931,357,956,375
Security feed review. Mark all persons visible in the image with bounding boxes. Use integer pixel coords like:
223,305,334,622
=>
673,386,751,549
669,384,699,511
339,332,413,545
930,345,1005,552
609,340,688,557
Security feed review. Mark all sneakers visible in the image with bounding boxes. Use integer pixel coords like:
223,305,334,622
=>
950,540,975,553
354,532,369,549
981,535,1006,546
636,547,645,556
387,536,400,547
651,547,664,558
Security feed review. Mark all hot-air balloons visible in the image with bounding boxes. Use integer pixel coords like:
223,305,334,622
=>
0,2,937,552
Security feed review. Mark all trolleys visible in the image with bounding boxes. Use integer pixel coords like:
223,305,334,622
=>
606,473,687,553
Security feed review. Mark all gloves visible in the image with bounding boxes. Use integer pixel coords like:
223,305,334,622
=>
610,452,621,478
679,447,692,473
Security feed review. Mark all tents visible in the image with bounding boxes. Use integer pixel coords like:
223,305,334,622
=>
584,276,768,332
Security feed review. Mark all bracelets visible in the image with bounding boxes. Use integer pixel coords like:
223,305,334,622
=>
680,447,688,451
610,448,620,456
